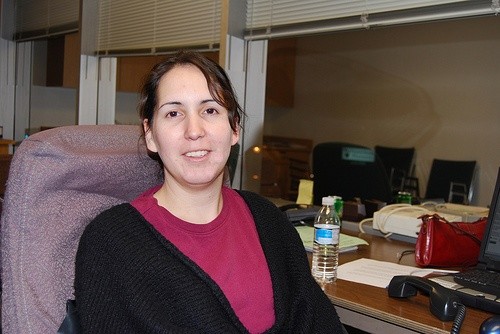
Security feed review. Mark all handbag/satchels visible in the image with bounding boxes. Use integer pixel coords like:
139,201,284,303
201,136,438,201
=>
415,214,488,267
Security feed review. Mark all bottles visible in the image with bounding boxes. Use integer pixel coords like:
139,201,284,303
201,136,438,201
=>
311,197,340,284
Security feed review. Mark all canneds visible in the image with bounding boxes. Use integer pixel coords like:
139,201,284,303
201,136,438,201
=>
327,196,343,232
396,192,412,204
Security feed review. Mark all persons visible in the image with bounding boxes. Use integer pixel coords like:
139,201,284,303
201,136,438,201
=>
73,50,343,334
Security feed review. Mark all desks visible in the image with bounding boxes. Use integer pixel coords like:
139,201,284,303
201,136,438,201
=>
293,226,492,334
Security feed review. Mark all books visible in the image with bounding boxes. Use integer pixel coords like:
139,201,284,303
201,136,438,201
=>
292,226,368,254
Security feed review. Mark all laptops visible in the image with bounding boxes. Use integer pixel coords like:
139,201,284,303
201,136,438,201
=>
427,165,500,315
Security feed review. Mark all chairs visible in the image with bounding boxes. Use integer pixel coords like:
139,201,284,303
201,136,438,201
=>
3,125,229,334
311,142,415,203
416,159,477,206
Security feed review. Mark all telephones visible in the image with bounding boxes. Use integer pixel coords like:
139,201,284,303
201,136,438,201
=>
277,203,325,221
388,275,457,321
